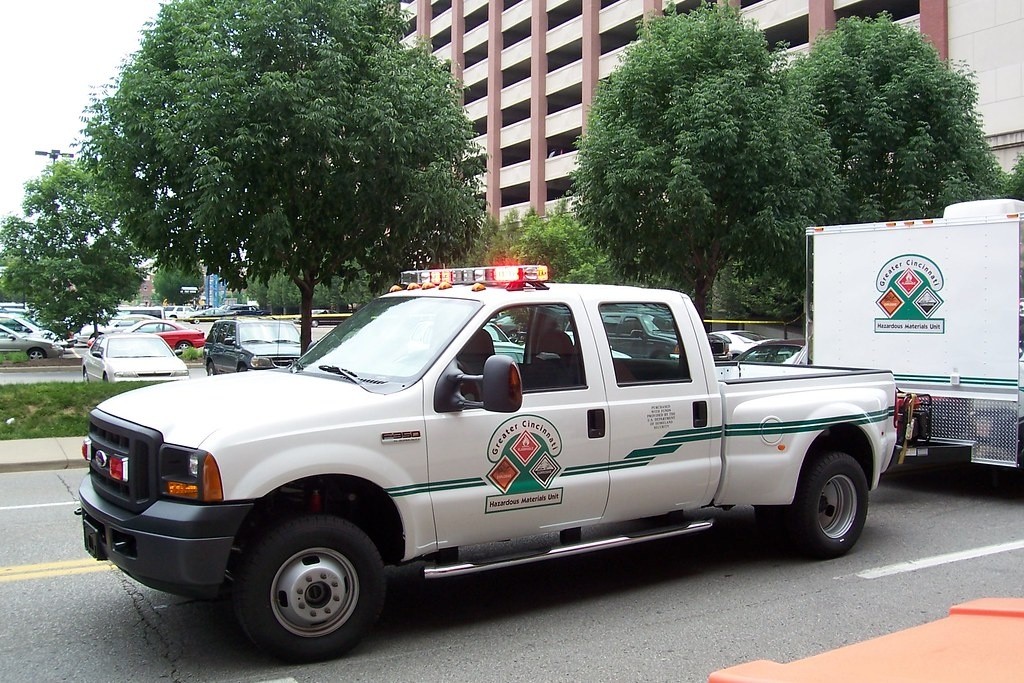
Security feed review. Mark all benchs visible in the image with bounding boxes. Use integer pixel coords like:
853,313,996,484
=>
613,358,676,382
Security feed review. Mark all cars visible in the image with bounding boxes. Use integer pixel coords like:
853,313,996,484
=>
183,309,236,324
218,304,271,316
81,333,191,385
87,320,206,351
196,304,212,311
708,330,769,358
293,310,343,327
0,314,64,360
77,315,161,343
0,303,45,328
165,306,196,319
728,339,809,363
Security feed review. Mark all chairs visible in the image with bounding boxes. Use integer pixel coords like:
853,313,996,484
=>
457,330,494,373
522,330,577,388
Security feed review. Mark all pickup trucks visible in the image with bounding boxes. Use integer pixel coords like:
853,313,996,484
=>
74,270,898,659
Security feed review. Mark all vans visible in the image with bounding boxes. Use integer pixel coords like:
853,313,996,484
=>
105,307,166,322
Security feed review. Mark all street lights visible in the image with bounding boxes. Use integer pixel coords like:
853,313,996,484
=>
33,148,73,325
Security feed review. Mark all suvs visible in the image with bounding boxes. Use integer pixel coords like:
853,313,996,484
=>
203,319,311,376
